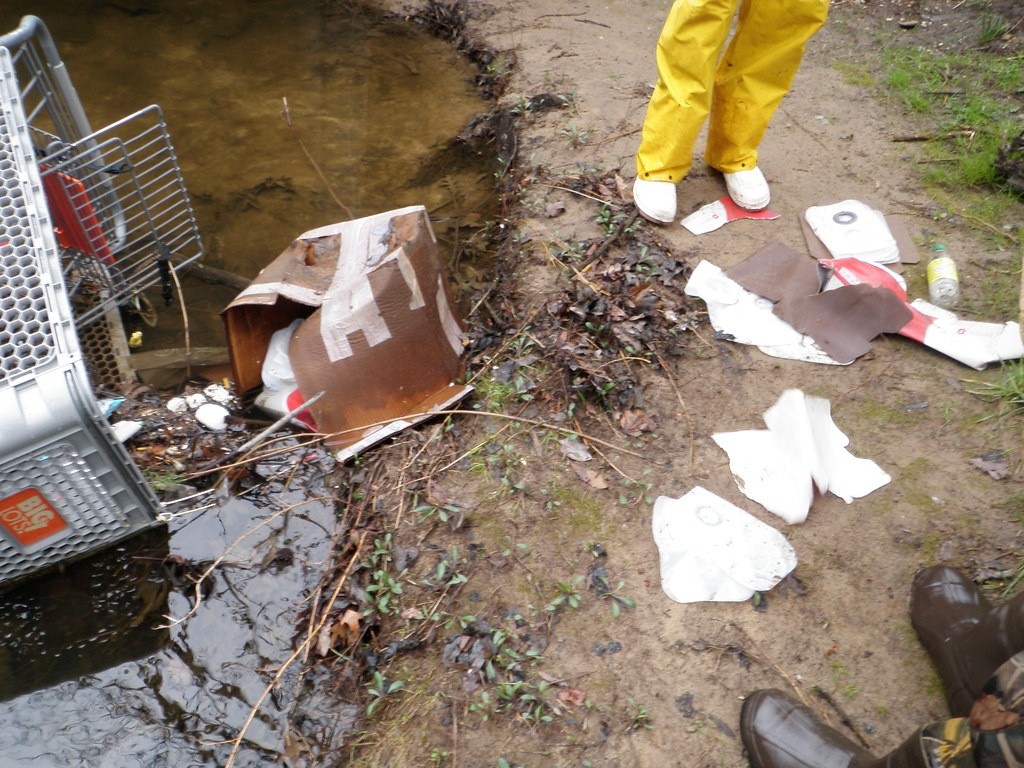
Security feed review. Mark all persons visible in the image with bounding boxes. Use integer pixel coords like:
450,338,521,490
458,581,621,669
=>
741,563,1023,768
633,0,830,224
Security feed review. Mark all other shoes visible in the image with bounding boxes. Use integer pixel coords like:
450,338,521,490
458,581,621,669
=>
632,176,677,226
723,165,770,212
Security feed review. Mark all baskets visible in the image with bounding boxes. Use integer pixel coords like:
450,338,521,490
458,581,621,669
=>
0,14,206,586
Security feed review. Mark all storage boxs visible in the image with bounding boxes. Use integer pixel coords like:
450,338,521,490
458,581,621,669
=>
220,205,475,464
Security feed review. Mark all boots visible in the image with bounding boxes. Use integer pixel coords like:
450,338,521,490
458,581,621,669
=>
908,563,1024,716
739,688,932,768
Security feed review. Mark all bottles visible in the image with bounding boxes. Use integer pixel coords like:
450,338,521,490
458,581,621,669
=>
261,318,304,389
926,244,959,310
